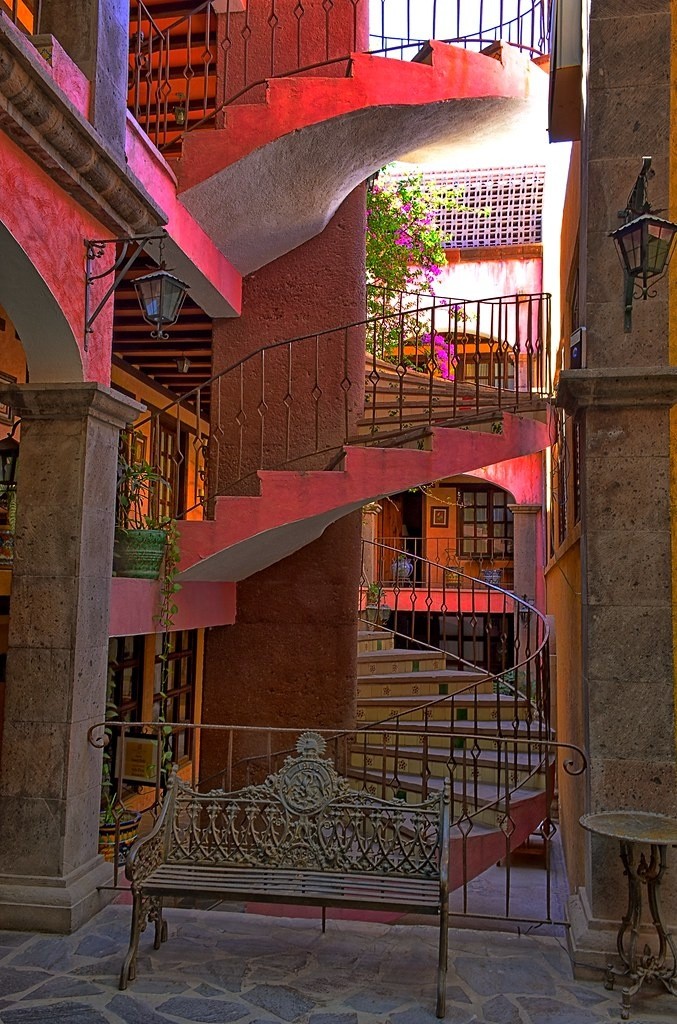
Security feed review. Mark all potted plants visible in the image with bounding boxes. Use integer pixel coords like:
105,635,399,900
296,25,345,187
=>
98,808,141,866
102,420,181,801
388,549,502,590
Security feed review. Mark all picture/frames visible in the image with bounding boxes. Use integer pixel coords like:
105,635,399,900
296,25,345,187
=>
429,505,448,528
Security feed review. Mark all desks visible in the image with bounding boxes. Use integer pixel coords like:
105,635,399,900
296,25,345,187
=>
465,551,514,590
578,807,676,1019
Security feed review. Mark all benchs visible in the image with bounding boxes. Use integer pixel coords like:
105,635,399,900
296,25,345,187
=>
116,728,452,1020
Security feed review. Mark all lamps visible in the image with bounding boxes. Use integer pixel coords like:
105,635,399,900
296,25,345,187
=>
0,419,21,490
82,231,191,349
608,155,676,332
